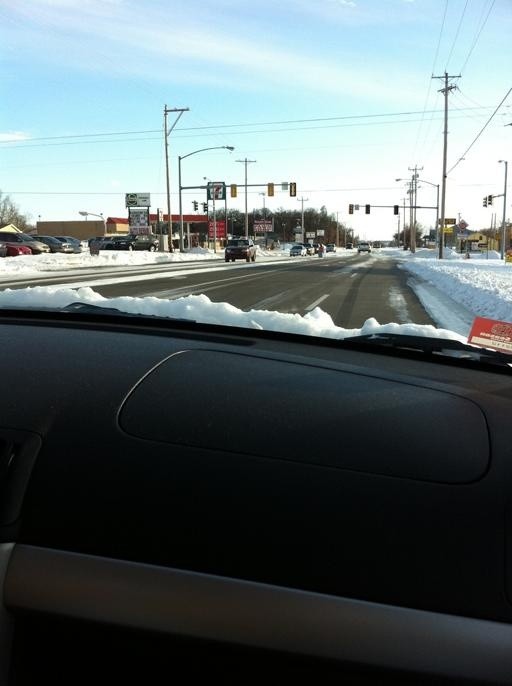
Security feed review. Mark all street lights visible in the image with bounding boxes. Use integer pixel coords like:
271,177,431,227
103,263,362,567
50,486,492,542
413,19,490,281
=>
80,212,108,236
205,177,217,253
179,146,234,252
396,178,439,255
498,159,507,259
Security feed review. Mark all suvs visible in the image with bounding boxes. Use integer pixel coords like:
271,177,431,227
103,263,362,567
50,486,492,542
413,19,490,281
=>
225,237,256,262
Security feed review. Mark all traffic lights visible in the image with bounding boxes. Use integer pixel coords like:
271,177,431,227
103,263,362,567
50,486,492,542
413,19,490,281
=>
483,195,493,207
192,201,208,213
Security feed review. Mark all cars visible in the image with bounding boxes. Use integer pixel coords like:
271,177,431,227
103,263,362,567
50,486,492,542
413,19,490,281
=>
374,243,381,248
345,242,354,249
357,242,371,252
290,243,335,257
0,231,80,257
88,234,158,252
